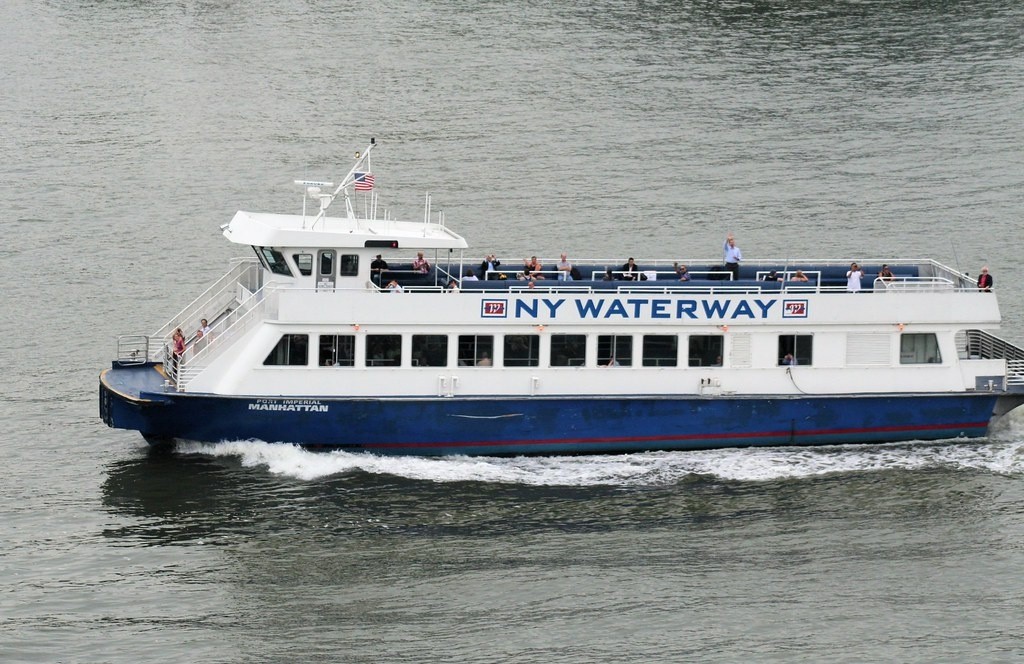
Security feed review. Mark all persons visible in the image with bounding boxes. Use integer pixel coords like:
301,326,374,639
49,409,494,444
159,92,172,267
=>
371,255,388,281
386,280,402,293
764,270,783,283
480,255,500,280
878,264,895,282
413,252,430,273
976,267,993,293
602,267,617,281
462,269,478,281
172,319,216,378
622,257,638,282
846,263,864,291
791,269,809,283
724,235,740,281
516,256,546,281
673,262,690,282
557,253,573,281
446,280,460,293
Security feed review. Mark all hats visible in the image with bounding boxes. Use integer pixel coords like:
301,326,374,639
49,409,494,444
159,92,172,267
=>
524,269,530,275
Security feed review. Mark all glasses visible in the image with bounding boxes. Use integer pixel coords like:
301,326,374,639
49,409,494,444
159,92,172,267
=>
680,268,684,270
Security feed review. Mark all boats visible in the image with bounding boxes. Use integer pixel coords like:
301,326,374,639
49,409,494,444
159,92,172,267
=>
98,137,1024,458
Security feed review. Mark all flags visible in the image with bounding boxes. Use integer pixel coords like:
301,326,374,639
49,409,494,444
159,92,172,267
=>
355,172,374,190
